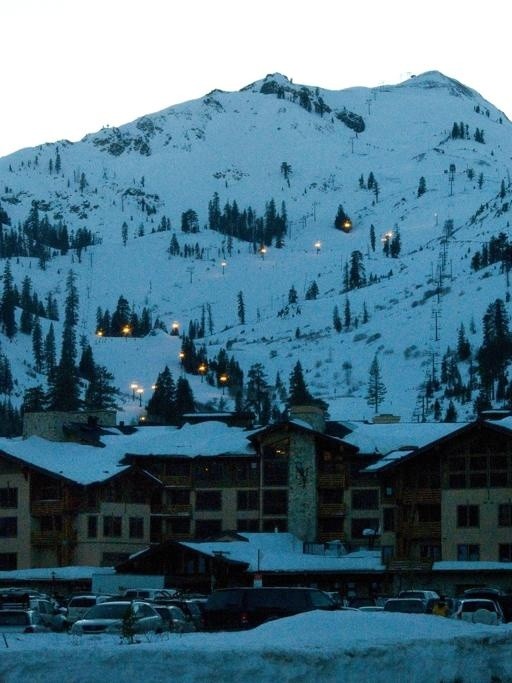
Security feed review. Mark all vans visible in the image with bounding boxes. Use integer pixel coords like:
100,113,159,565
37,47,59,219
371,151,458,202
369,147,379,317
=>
205,585,361,633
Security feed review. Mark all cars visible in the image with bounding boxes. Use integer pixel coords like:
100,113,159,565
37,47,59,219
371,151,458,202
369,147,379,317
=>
382,587,512,627
1,587,204,636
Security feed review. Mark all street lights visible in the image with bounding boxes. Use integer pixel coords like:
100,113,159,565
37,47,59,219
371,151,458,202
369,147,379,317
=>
95,320,230,426
221,221,393,274
